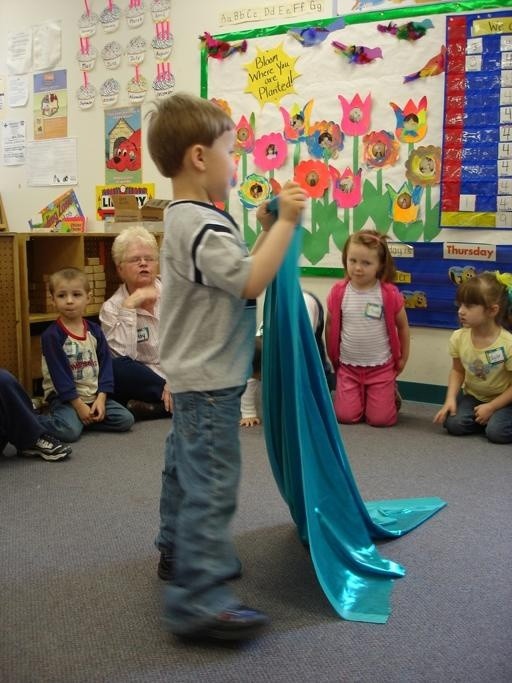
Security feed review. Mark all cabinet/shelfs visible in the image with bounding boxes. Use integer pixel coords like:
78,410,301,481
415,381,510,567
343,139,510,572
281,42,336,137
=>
15,229,164,403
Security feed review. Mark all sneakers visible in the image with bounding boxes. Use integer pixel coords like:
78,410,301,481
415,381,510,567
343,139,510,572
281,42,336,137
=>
394,389,401,412
157,554,240,581
172,606,270,639
16,433,71,461
126,399,153,421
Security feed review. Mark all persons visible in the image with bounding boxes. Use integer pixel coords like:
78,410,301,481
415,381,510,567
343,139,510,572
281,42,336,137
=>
32,267,136,443
1,367,73,461
98,225,173,422
324,229,411,428
144,92,309,644
432,273,512,444
240,288,333,428
